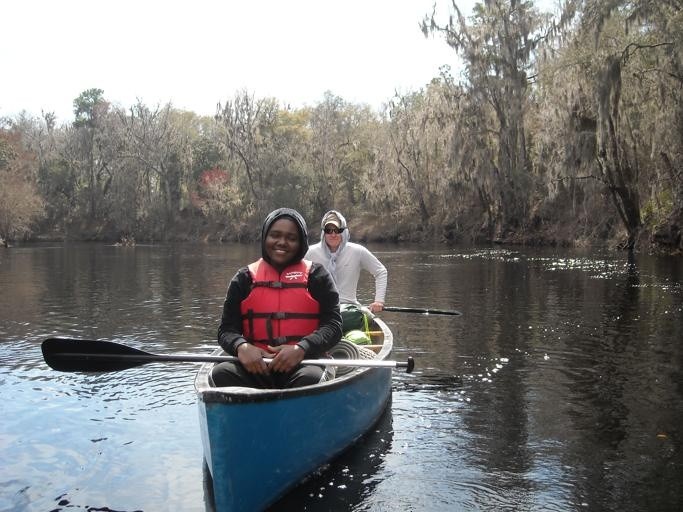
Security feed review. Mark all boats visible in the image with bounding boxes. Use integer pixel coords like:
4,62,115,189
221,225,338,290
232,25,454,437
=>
193,304,396,512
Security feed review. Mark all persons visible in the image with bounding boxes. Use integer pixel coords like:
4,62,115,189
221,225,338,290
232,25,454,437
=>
213,208,342,390
302,211,387,336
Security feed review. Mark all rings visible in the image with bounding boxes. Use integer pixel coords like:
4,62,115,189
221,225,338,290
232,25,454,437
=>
278,362,282,365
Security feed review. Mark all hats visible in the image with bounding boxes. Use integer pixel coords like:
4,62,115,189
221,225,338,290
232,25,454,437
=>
323,213,341,230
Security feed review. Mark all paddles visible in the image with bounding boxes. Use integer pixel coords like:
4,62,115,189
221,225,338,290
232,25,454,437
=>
342,304,461,315
40,338,414,373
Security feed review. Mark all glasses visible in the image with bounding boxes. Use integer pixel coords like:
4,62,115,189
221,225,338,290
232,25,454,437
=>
324,227,345,234
